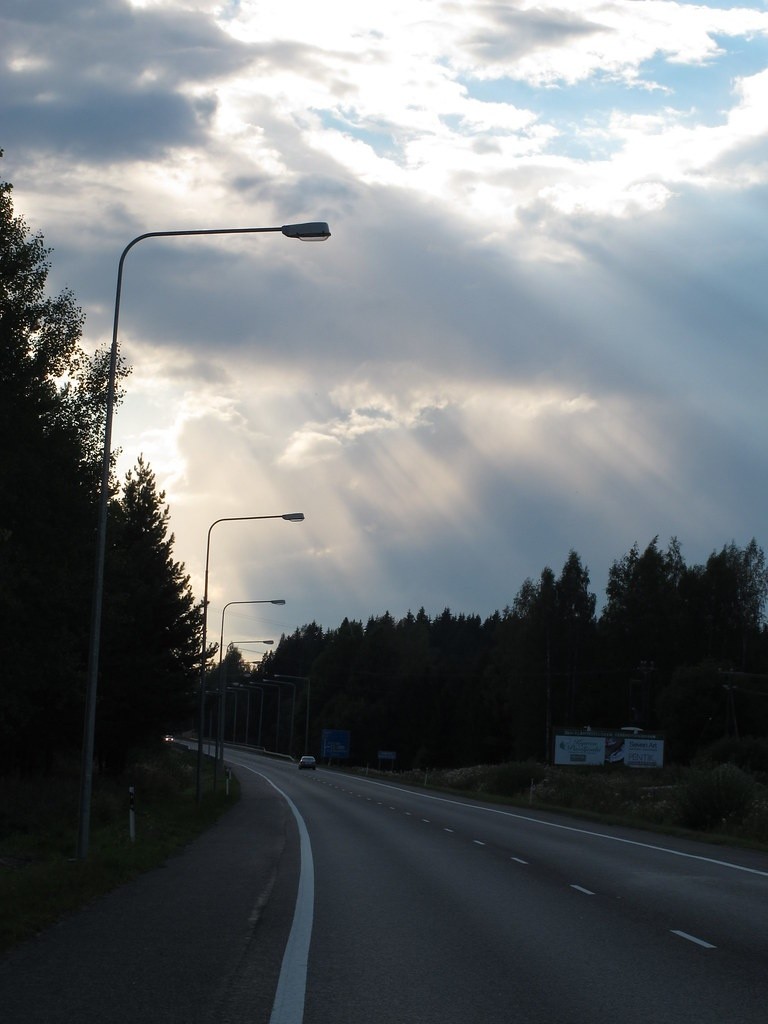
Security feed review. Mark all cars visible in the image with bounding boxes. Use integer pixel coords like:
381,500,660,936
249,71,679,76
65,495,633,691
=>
298,755,316,771
165,734,174,743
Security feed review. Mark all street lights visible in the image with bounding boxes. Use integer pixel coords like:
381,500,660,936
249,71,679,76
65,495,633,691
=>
73,220,332,862
182,674,312,764
189,512,307,807
209,599,286,793
217,639,274,781
